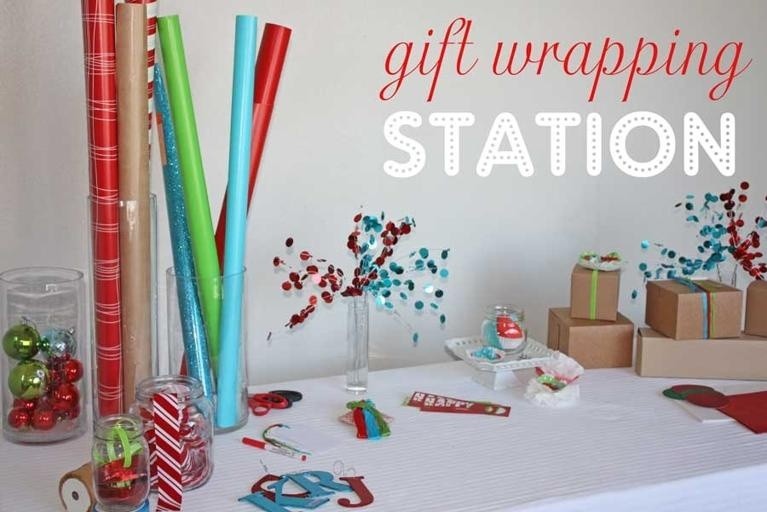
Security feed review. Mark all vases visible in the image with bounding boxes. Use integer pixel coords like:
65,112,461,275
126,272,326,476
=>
347,297,370,392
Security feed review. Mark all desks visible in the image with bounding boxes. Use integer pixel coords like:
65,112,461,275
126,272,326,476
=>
1,357,766,511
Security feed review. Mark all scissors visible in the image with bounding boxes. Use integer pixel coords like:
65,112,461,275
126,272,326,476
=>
247,390,302,409
247,393,289,416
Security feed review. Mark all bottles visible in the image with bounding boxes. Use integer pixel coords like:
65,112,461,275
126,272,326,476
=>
481,304,530,353
0,194,248,447
92,376,215,512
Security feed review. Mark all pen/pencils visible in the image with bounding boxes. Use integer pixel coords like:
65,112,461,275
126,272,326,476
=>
242,437,306,461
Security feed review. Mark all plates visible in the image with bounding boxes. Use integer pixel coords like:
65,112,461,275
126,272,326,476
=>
465,348,506,364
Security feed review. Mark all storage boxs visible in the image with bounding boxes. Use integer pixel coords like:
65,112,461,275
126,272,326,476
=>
546,251,767,379
